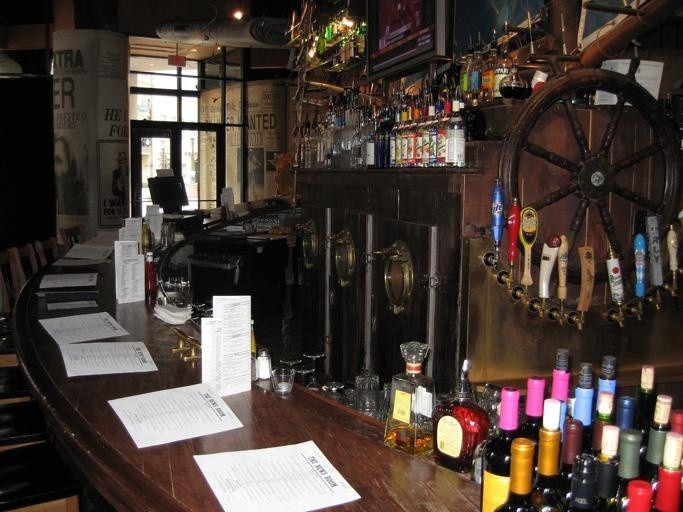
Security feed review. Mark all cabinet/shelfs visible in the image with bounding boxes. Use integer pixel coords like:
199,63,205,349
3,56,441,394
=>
296,37,680,402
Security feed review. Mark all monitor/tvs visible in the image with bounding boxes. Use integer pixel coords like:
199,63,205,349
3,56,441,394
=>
149,179,192,216
365,0,450,83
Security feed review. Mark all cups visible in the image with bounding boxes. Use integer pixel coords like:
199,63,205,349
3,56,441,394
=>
162,223,175,246
343,391,356,407
270,369,295,395
355,375,378,410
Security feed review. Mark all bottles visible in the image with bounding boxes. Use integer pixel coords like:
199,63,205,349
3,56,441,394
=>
145,252,157,305
251,320,256,352
295,26,513,168
479,347,682,508
379,383,391,418
251,352,257,382
144,219,151,243
257,349,272,379
433,373,489,473
383,342,436,457
142,223,149,247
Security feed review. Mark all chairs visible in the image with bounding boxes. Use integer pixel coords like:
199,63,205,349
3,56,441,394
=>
35,240,48,269
78,222,87,246
59,226,72,257
0,400,54,448
26,244,37,276
0,267,18,326
1,442,82,511
7,245,29,291
2,365,35,401
48,236,62,263
1,331,22,358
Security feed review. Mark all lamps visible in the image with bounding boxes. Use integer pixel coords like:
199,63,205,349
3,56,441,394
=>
167,40,186,66
